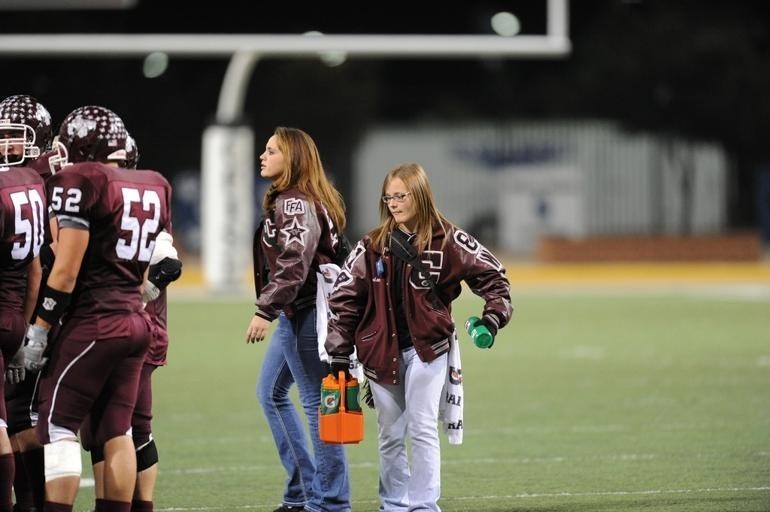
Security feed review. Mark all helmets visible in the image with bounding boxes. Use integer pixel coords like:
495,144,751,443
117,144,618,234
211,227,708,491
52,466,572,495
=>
0,94,140,176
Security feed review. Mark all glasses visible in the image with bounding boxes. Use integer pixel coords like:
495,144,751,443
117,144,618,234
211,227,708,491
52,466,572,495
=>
381,191,411,204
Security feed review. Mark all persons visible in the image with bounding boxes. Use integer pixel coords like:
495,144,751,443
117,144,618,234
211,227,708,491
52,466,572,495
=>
0,94,182,511
321,161,513,512
242,126,358,512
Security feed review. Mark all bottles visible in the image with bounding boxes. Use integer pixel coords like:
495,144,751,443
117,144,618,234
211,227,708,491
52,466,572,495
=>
318,374,360,414
465,317,494,349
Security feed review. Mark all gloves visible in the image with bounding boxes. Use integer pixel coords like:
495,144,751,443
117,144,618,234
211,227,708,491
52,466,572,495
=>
2,323,49,384
331,361,350,382
474,317,496,349
